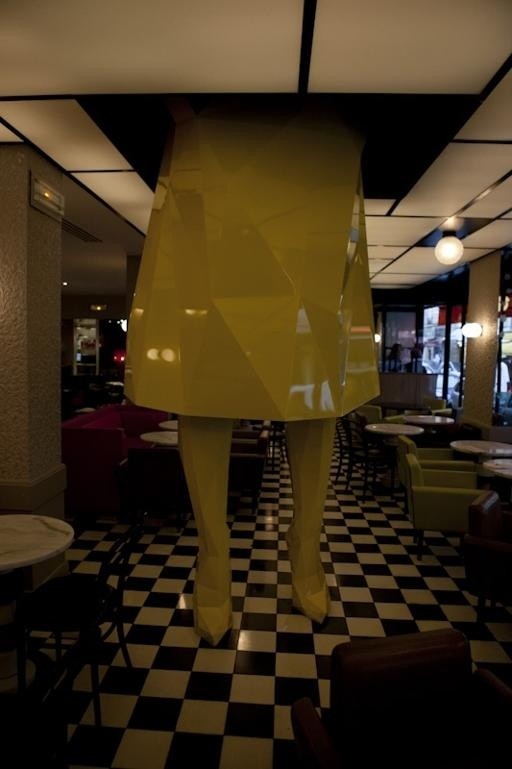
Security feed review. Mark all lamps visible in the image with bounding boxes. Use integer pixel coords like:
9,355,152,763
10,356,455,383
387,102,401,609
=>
435,230,464,265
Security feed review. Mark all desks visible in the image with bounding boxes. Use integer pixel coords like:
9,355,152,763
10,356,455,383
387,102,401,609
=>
0,513,75,573
160,418,179,430
140,431,179,446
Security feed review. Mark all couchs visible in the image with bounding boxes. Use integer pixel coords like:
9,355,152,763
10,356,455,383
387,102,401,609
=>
62,404,170,515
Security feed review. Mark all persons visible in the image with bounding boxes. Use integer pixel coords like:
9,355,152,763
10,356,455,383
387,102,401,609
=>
122,104,381,649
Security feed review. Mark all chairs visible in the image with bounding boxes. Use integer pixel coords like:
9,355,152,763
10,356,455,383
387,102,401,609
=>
228,420,289,513
14,511,150,739
